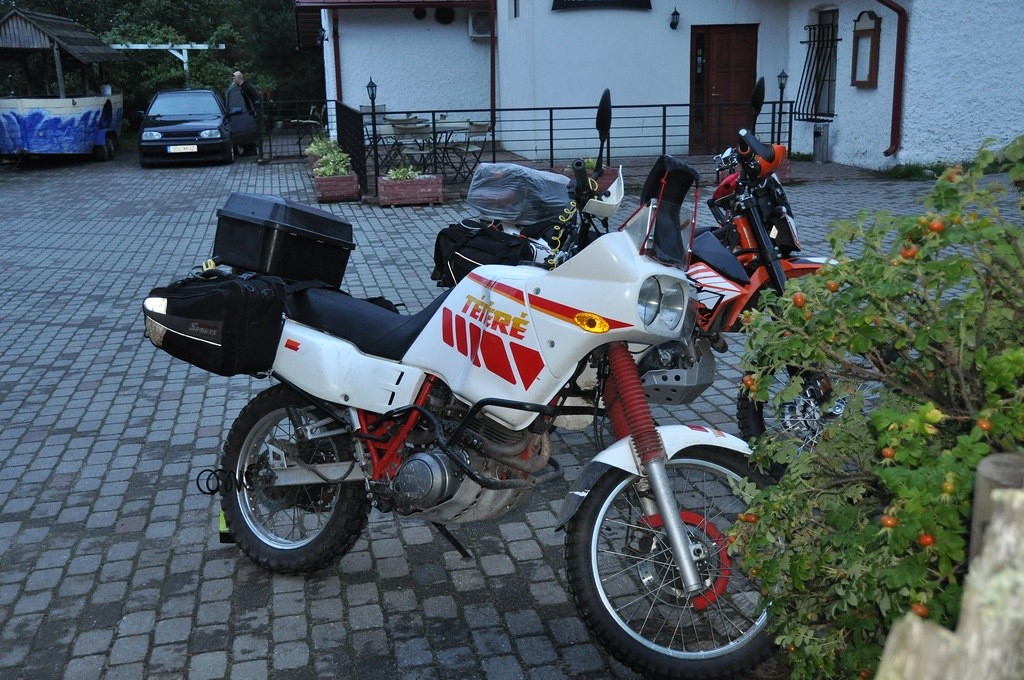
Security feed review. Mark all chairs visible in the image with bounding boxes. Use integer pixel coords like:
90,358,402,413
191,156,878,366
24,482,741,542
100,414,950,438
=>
360,104,495,178
290,103,327,145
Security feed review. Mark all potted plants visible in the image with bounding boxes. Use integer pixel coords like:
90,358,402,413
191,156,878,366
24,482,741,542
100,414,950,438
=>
377,165,443,208
564,156,619,195
304,135,359,203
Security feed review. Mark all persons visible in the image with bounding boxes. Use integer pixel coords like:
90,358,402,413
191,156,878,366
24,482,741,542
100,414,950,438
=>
233,71,263,156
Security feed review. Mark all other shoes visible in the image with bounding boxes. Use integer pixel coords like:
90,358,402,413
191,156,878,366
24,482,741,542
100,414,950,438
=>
239,150,256,156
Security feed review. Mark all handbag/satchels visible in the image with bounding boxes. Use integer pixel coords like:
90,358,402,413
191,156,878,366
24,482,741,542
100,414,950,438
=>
432,219,533,288
142,262,284,378
361,296,407,313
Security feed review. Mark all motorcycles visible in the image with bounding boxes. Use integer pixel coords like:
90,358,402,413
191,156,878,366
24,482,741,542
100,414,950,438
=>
141,89,792,680
431,76,898,479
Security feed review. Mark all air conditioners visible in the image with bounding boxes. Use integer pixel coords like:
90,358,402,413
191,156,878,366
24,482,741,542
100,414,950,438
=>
468,9,497,41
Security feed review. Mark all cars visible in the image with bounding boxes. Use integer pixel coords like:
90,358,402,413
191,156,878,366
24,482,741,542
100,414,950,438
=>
135,88,260,167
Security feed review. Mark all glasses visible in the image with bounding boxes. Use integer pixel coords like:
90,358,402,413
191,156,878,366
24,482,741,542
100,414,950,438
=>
232,74,240,79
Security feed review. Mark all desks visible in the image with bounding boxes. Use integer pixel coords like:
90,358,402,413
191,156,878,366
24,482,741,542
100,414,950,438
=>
392,125,467,181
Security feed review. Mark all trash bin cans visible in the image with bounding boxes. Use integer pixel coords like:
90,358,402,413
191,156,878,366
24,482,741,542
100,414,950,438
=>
813,124,830,165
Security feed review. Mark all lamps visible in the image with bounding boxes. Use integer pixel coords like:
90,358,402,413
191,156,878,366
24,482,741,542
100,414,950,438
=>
777,70,789,90
669,7,680,31
316,25,328,42
365,76,377,100
